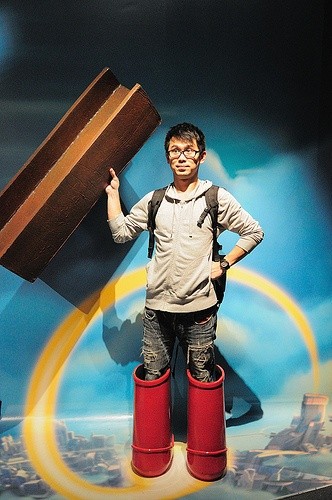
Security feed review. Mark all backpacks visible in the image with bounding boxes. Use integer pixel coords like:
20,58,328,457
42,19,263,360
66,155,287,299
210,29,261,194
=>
148,186,226,304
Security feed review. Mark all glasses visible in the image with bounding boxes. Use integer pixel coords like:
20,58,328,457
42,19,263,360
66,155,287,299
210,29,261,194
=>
168,148,200,159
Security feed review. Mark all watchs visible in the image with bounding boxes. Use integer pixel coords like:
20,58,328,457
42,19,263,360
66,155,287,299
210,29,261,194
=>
218,258,231,270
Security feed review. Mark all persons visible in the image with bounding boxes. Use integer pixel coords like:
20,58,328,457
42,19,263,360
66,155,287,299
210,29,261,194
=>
104,123,264,384
